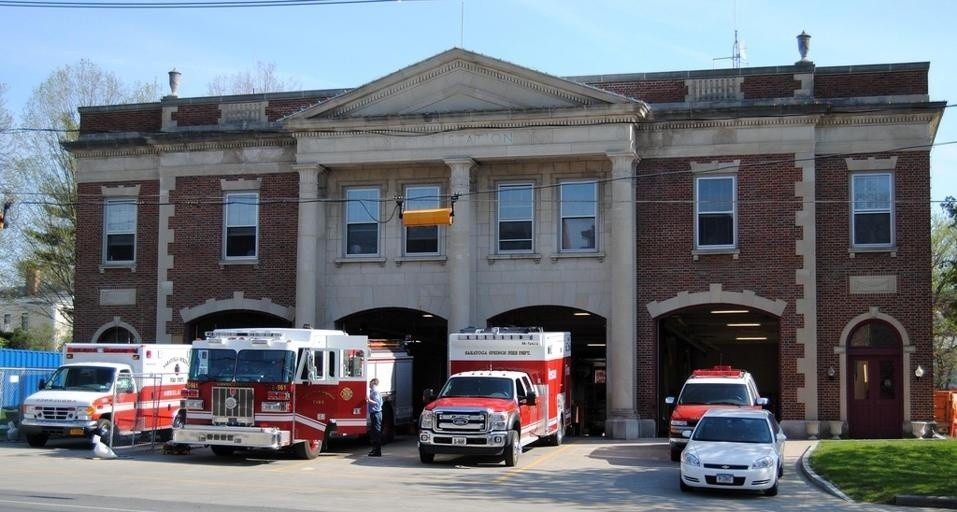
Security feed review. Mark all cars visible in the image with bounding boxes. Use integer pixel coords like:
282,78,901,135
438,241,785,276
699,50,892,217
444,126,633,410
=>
664,365,787,496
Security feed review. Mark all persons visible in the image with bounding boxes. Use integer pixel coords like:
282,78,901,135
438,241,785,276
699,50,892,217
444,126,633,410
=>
365,378,383,457
491,383,508,398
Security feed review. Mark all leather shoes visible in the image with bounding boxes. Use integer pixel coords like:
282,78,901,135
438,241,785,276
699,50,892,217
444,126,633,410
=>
367,450,382,457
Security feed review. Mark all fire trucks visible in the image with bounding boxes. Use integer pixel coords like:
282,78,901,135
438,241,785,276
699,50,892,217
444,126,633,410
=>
419,326,587,466
19,344,195,454
172,327,416,461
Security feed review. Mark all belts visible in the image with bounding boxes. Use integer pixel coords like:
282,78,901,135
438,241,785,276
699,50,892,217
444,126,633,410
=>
371,410,383,412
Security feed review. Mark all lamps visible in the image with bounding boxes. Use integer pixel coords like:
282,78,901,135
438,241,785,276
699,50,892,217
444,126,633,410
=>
828,366,836,381
915,365,925,384
